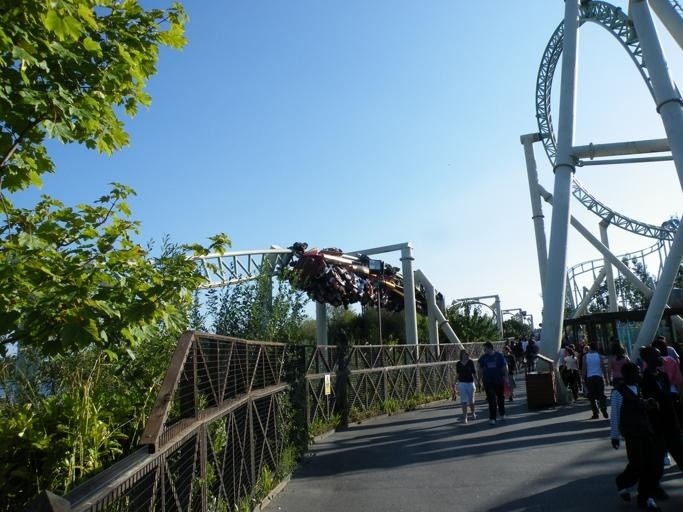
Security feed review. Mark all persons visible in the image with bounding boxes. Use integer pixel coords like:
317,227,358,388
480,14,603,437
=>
511,336,541,373
477,341,509,424
559,336,682,511
452,349,478,424
502,346,515,401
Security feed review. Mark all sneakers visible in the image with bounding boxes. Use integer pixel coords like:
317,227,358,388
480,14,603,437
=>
463,413,506,425
591,407,608,419
615,476,632,502
637,482,670,512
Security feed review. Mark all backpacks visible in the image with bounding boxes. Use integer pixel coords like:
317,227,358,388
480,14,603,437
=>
614,383,662,442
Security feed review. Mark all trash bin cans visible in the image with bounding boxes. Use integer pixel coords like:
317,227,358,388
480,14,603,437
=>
525,353,557,409
